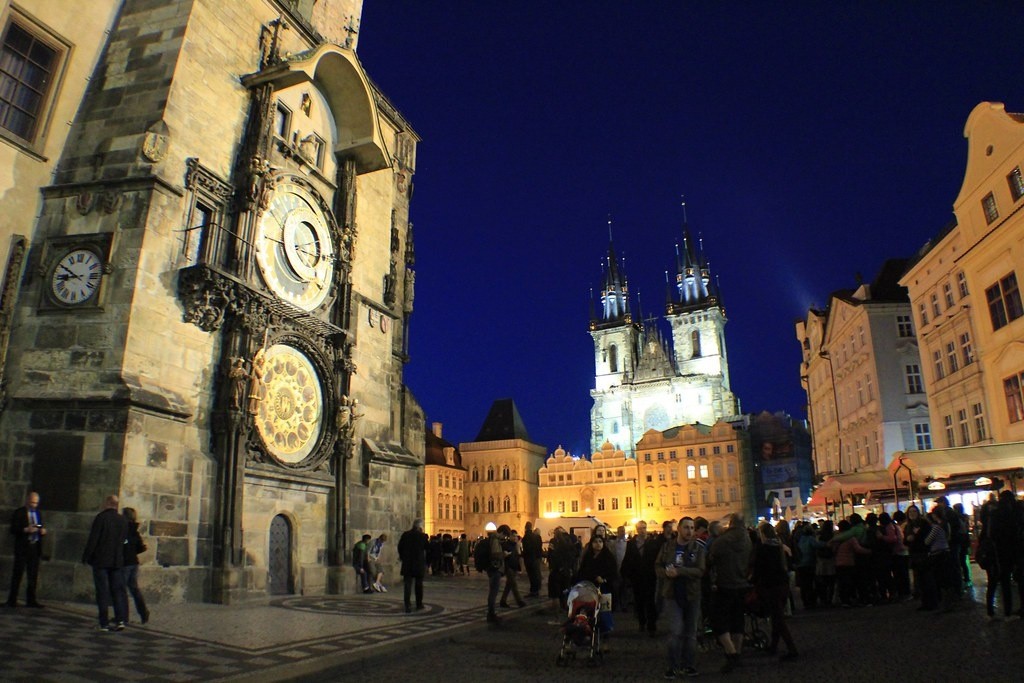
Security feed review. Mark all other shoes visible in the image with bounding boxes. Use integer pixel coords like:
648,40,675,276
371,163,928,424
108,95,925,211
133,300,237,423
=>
760,646,778,654
779,652,800,662
24,602,47,609
421,566,470,576
363,586,373,593
4,603,22,608
696,635,768,652
783,578,1023,622
405,605,425,612
638,625,655,639
518,600,526,607
523,591,540,598
111,617,129,624
141,610,150,624
719,656,743,671
500,599,510,608
617,605,630,613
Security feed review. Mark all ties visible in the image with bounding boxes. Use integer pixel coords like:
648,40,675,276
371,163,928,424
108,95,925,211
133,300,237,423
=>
31,509,35,542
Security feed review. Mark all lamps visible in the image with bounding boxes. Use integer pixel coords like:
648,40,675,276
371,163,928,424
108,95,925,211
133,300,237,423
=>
974,477,993,486
927,482,946,491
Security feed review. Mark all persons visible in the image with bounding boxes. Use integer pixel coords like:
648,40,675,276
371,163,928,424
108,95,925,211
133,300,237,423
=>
120,507,150,626
777,490,1024,621
753,522,802,664
424,515,777,637
0,491,47,610
335,394,365,441
569,536,618,655
248,157,277,211
706,513,753,671
366,534,387,593
229,356,265,418
398,519,430,613
655,516,707,680
82,493,129,632
353,534,374,594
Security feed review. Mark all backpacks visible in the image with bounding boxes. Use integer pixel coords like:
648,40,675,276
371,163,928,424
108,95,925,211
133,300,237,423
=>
975,532,993,571
473,538,490,573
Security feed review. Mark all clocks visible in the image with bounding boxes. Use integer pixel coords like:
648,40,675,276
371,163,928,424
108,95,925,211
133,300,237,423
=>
44,242,106,308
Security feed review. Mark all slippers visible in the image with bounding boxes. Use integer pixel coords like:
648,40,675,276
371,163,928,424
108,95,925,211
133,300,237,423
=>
547,619,564,626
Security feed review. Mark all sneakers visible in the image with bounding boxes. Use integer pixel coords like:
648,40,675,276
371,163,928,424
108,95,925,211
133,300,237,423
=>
664,666,698,681
373,582,387,592
95,620,125,633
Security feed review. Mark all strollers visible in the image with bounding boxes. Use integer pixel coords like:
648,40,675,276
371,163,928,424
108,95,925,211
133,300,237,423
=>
695,569,771,654
550,575,607,669
